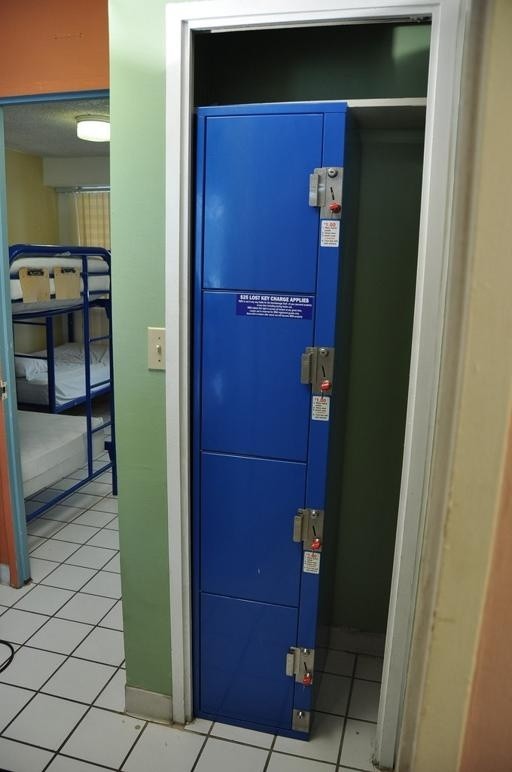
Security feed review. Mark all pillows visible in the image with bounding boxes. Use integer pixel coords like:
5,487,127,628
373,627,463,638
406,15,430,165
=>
15,352,46,380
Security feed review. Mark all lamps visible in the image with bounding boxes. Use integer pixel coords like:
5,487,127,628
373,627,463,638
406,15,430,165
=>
75,114,110,143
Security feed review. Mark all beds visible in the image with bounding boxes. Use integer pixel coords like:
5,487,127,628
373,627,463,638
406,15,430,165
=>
8,243,117,524
12,316,110,413
